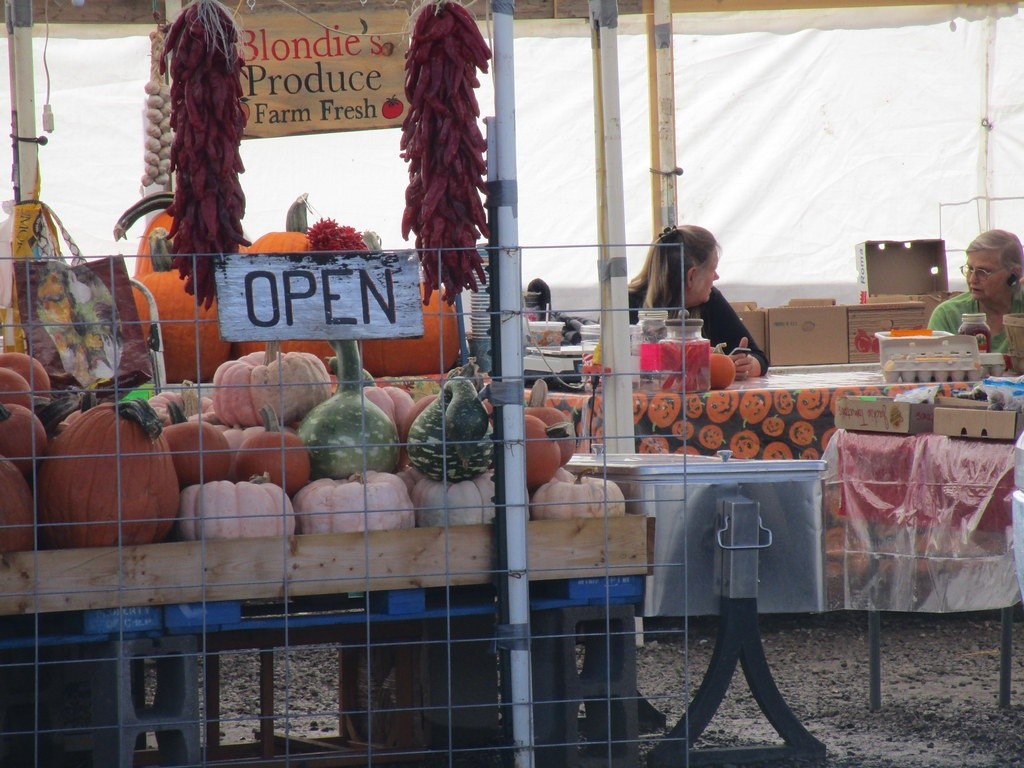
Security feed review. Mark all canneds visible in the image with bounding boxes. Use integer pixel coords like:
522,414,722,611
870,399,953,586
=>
958,313,991,353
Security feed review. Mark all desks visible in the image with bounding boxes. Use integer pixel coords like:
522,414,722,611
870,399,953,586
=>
525,372,982,595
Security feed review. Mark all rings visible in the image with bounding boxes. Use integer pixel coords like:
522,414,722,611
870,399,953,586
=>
746,370,749,376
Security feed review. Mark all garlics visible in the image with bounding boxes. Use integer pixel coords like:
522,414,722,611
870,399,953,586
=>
140,81,175,187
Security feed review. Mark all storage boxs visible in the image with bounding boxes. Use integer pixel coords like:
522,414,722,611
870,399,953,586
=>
727,238,965,367
629,448,826,617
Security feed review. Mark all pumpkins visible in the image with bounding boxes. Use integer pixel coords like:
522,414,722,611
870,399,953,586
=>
708,342,736,389
0,193,624,554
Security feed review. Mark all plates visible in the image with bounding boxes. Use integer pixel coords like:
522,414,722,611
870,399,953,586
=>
470,242,491,341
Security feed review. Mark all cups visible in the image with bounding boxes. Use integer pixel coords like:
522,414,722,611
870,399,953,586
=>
529,321,565,347
580,324,640,394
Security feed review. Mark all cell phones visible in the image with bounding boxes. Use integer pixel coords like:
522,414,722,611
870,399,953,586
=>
730,348,752,356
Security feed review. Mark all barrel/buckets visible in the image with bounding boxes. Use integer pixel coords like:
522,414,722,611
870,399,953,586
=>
1002,314,1024,373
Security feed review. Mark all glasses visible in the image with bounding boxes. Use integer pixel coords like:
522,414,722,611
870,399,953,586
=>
960,264,1005,282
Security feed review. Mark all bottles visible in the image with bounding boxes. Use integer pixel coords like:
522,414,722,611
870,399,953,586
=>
959,313,991,353
659,319,710,393
636,311,668,392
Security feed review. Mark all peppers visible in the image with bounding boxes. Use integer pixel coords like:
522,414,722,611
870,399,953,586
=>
160,0,252,310
399,0,494,306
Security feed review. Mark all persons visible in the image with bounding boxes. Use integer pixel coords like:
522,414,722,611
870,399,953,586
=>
627,223,772,385
922,227,1024,363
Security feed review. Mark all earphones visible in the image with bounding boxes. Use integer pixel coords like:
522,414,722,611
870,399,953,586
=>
1008,273,1018,286
